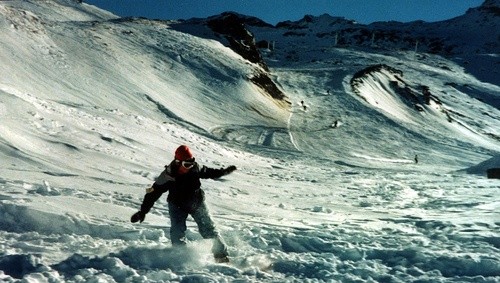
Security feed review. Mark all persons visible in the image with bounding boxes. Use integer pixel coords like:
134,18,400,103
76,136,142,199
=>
130,144,238,263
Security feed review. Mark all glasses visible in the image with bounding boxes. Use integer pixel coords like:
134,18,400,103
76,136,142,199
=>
175,159,195,169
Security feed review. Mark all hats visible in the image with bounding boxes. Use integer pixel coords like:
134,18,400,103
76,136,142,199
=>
173,145,193,160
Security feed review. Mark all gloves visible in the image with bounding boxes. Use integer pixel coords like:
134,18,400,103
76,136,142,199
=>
224,165,237,176
130,211,145,224
